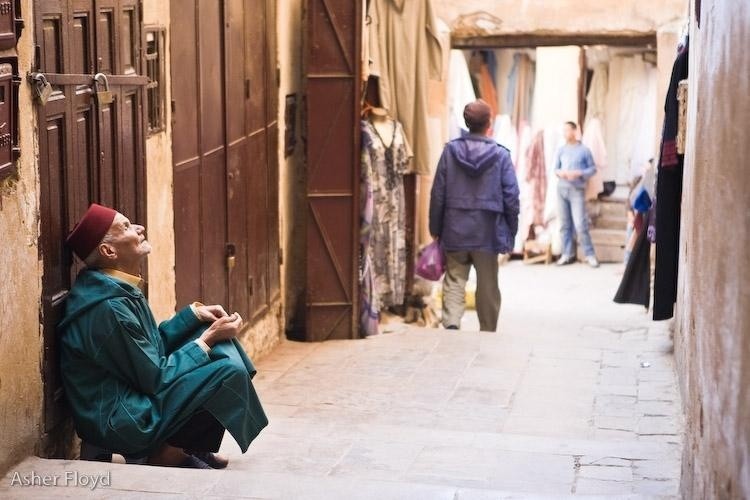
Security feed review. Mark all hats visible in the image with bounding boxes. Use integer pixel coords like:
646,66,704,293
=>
66,204,116,260
464,102,490,123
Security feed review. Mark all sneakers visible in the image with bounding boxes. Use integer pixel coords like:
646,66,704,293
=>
586,256,598,268
558,255,574,265
180,447,228,469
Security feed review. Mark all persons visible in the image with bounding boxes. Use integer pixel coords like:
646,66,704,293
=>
621,175,642,270
428,101,520,332
61,204,268,469
361,107,414,308
554,123,600,267
